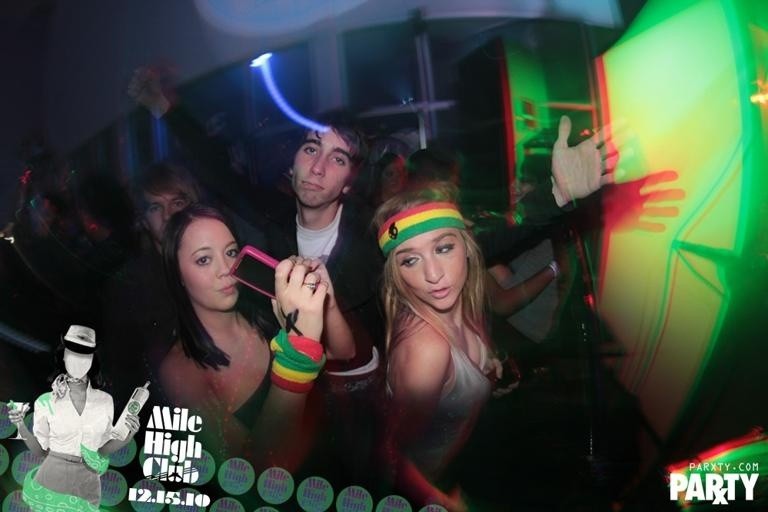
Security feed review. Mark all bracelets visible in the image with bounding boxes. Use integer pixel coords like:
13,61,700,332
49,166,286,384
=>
269,332,328,395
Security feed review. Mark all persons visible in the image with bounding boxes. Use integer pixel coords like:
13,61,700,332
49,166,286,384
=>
102,64,642,512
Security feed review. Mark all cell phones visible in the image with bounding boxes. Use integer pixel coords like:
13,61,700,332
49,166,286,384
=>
230,245,292,301
